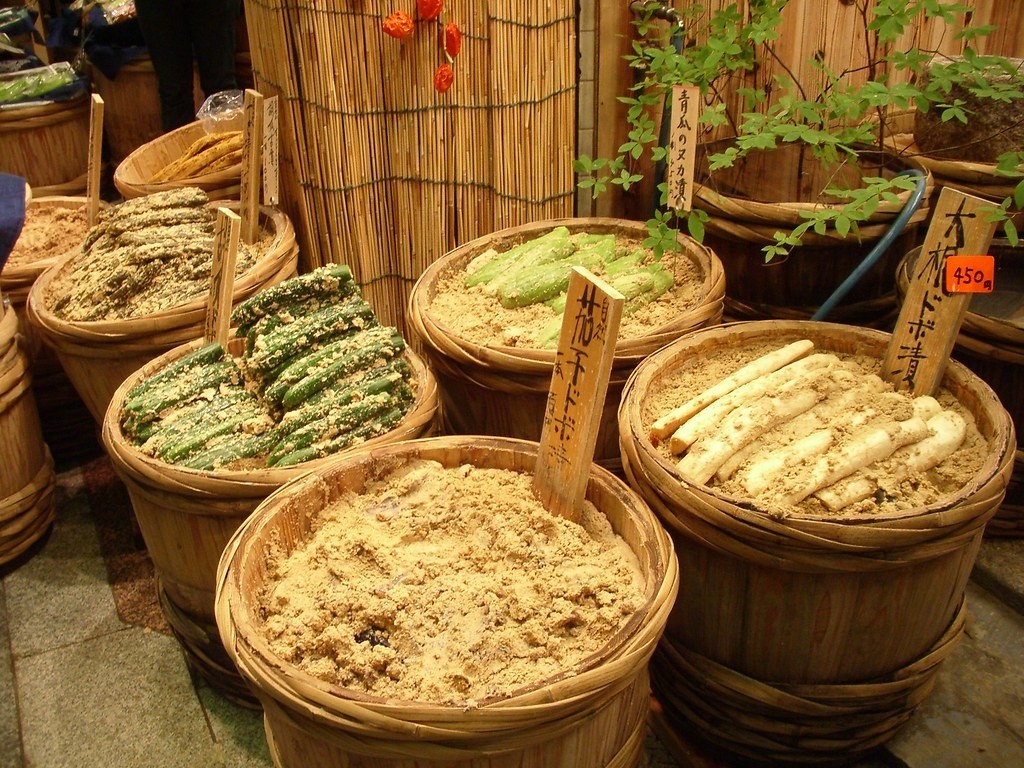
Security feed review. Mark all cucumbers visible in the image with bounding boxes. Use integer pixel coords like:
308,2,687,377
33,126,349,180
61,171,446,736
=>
464,227,674,349
120,263,412,473
